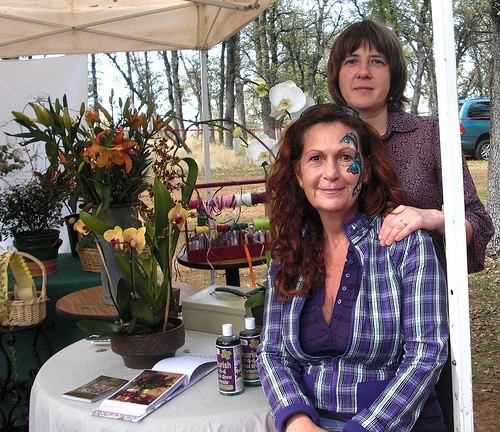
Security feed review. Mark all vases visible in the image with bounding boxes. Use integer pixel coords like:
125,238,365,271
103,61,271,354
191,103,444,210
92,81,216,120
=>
245,287,265,325
109,316,185,369
91,201,144,306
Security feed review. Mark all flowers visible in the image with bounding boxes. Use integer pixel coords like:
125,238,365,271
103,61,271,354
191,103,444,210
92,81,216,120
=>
5,92,177,219
72,157,198,338
179,70,316,310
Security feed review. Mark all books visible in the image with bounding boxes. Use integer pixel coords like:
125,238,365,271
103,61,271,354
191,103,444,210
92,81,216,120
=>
62,374,129,403
89,354,218,424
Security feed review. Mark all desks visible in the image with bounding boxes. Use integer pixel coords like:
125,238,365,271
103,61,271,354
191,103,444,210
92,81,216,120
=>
56,279,201,323
177,246,269,287
29,317,280,432
2,252,103,391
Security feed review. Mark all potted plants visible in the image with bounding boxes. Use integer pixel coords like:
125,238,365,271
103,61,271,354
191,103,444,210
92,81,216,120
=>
0,168,76,277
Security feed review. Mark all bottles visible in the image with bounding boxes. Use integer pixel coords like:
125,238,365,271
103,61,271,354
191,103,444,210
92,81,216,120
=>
215,323,244,396
187,220,272,248
239,318,263,387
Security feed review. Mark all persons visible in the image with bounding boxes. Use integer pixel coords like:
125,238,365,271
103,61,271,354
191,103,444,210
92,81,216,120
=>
255,103,450,432
327,20,495,432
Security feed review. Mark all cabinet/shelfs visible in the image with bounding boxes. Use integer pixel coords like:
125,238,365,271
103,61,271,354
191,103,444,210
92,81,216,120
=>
1,314,47,432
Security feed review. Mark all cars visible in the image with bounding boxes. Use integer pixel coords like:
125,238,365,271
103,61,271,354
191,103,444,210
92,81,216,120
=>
432,100,463,118
458,98,490,161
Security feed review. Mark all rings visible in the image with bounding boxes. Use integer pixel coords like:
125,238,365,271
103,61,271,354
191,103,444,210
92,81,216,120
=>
401,220,407,226
